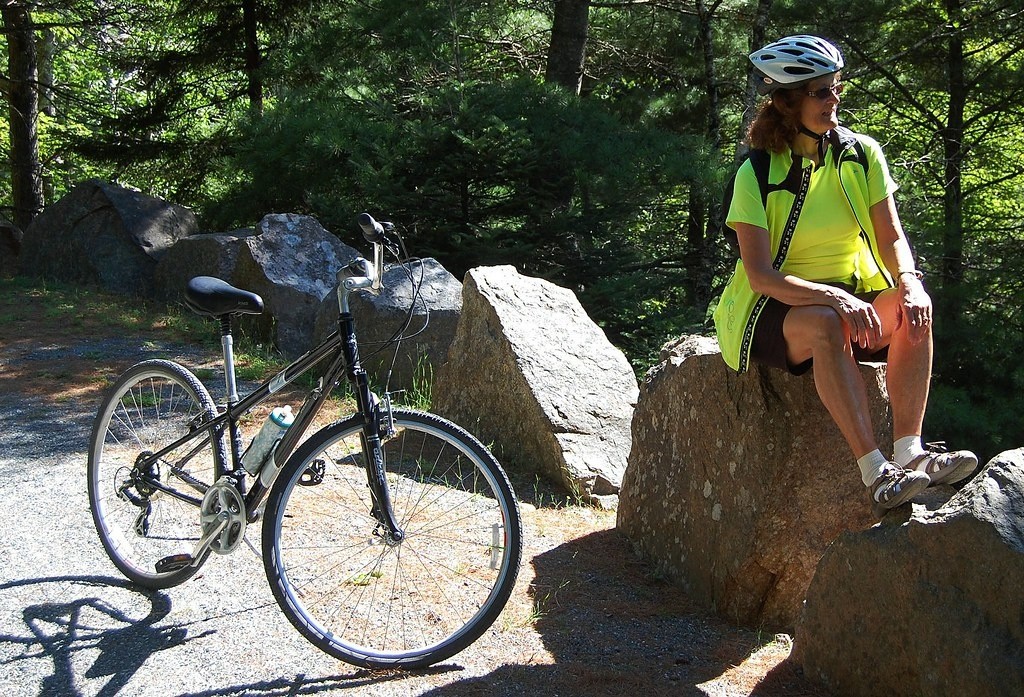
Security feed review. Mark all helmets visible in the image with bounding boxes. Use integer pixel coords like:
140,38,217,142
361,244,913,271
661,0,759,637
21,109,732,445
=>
749,35,844,96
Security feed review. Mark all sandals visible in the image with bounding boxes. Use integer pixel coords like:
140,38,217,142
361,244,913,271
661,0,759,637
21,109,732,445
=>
898,441,978,487
866,461,930,519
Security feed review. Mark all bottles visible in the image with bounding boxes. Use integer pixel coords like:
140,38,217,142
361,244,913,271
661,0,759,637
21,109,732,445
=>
243,405,296,474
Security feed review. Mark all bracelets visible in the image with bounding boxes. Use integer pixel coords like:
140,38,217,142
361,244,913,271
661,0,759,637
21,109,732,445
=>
897,271,923,287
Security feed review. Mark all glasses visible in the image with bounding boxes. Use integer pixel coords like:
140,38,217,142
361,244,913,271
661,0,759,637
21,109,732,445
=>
803,82,843,100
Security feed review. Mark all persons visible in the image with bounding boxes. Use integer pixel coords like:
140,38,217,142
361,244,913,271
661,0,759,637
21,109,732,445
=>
711,34,980,510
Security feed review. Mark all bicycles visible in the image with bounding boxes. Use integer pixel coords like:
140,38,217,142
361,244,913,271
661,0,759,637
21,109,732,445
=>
84,210,525,673
